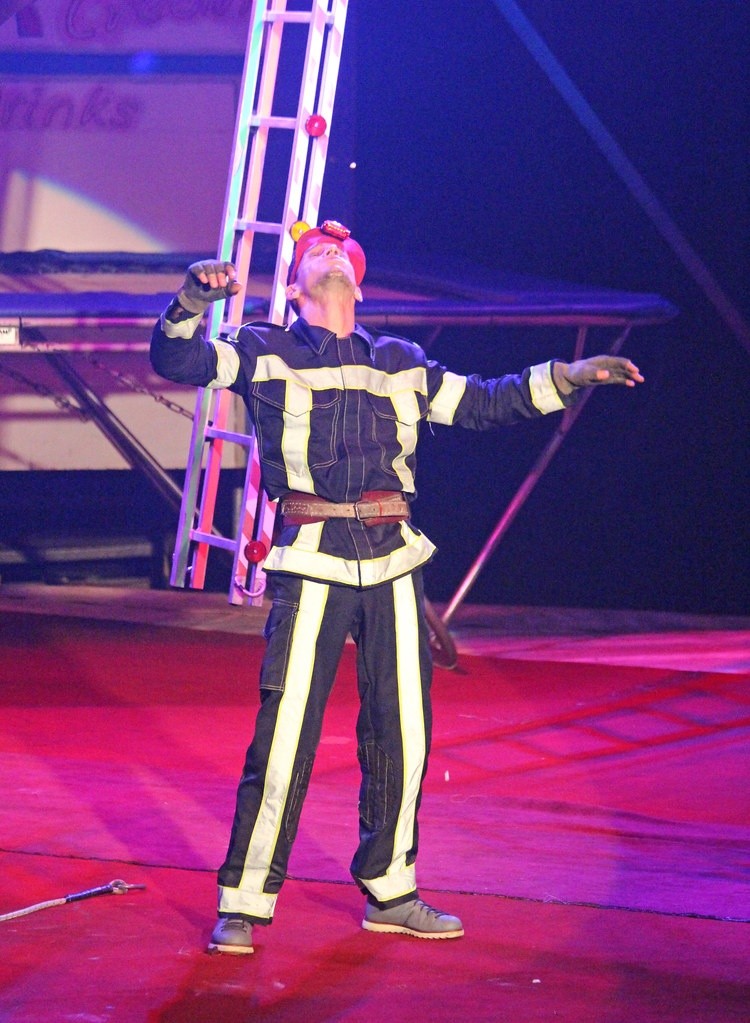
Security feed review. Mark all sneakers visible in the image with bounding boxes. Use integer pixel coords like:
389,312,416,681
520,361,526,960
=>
208,917,254,954
362,894,464,939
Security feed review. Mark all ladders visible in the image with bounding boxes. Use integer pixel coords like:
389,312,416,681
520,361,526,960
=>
168,0,353,608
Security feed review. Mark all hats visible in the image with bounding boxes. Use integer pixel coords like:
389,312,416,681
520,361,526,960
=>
286,227,366,317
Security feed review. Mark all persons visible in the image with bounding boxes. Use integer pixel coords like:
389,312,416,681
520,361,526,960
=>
149,220,645,954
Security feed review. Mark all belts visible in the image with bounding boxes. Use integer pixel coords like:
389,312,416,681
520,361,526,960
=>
281,494,408,521
